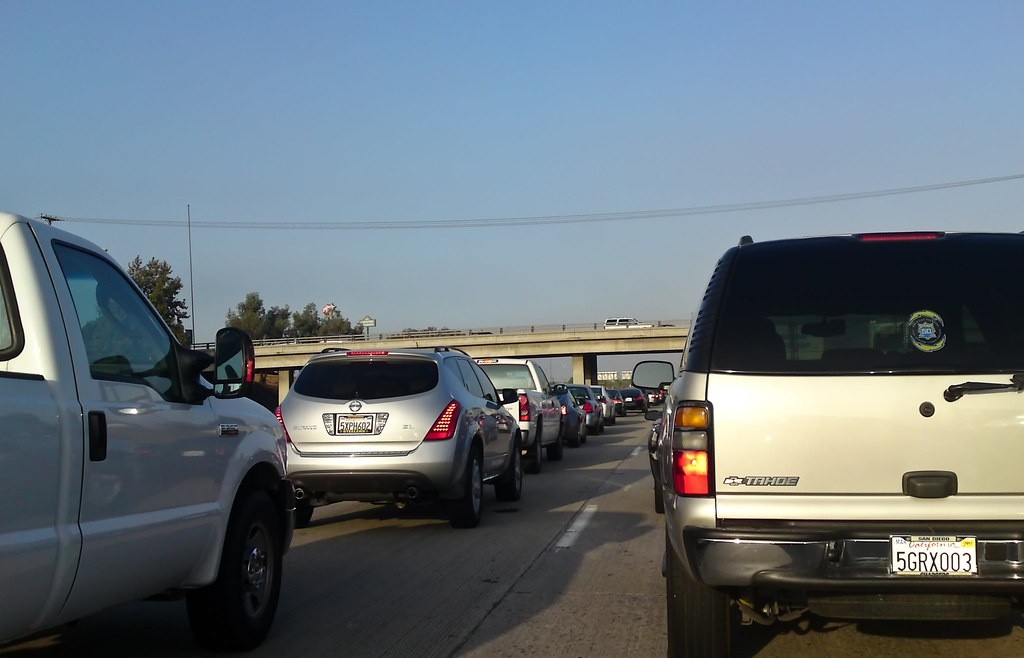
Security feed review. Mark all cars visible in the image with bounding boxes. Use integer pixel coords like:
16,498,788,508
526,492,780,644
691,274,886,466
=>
551,386,587,448
607,389,667,416
642,411,665,512
590,385,615,425
551,384,604,435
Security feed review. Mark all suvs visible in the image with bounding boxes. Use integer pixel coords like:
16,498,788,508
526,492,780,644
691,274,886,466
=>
632,235,1024,658
604,318,654,329
274,346,523,528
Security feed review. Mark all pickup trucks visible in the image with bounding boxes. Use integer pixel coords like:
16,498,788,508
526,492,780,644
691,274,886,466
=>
0,212,288,653
473,359,568,475
